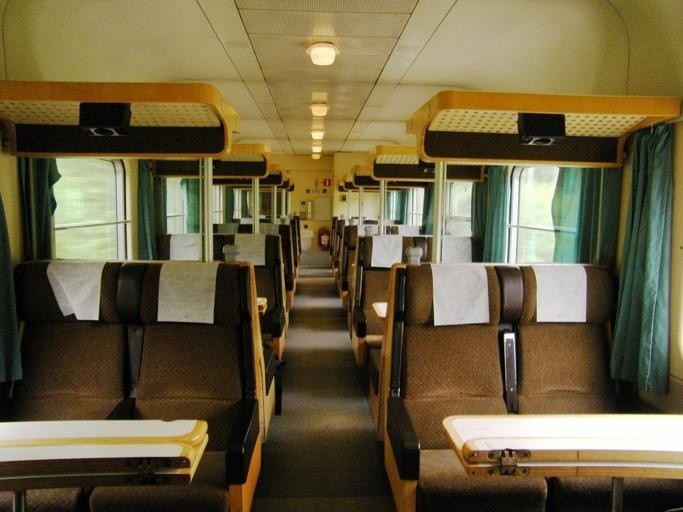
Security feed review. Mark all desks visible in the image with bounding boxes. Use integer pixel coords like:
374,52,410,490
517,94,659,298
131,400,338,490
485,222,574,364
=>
442,413,683,480
3,416,210,510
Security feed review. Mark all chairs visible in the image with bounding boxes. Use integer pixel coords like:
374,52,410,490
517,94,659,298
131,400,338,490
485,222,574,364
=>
375,261,546,510
512,260,680,507
331,216,494,424
159,216,304,439
2,260,129,509
90,262,262,512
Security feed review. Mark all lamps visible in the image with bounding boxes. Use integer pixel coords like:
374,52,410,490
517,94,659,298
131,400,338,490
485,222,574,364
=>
304,42,340,66
307,103,330,116
309,128,325,139
